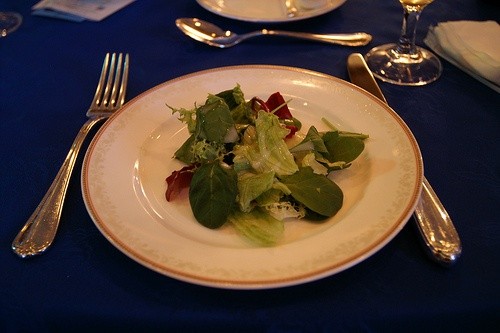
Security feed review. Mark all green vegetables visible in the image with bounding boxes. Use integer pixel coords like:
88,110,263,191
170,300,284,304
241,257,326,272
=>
167,83,371,248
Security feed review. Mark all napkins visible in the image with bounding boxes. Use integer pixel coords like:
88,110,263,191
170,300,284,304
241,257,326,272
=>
424,19,500,92
